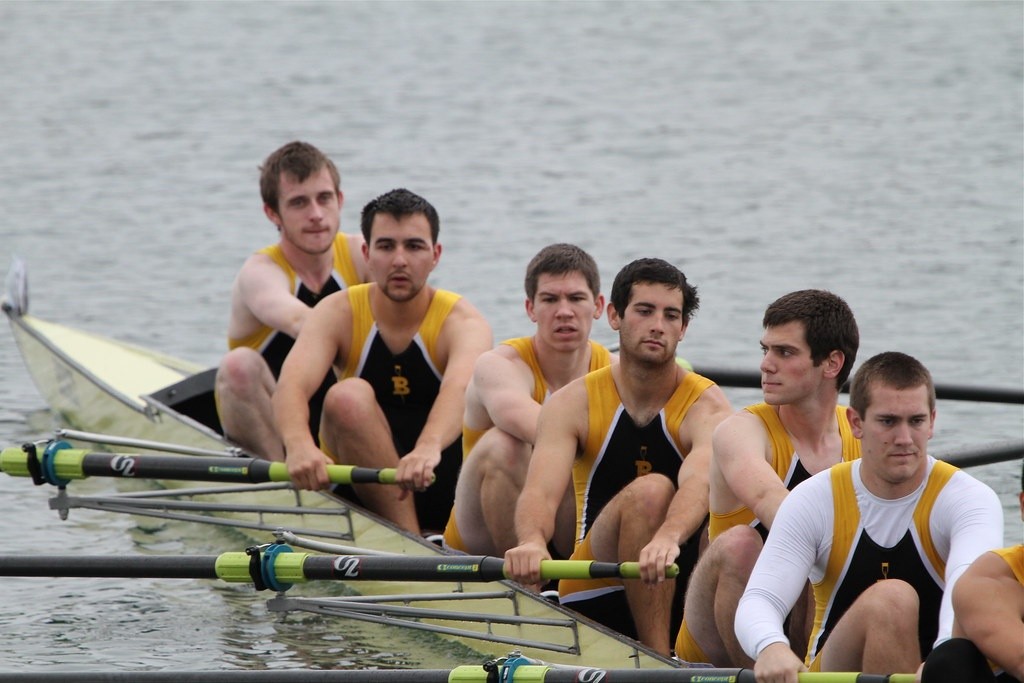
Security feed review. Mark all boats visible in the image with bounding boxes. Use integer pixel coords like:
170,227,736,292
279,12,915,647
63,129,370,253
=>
2,302,696,683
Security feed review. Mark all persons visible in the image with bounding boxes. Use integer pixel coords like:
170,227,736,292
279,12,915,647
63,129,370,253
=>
441,242,618,559
502,257,733,657
213,140,376,462
921,458,1024,682
673,288,862,668
733,351,1004,682
271,187,493,536
